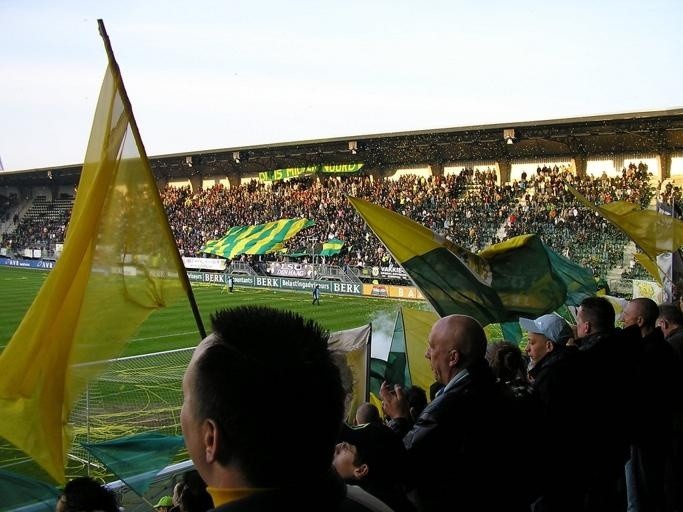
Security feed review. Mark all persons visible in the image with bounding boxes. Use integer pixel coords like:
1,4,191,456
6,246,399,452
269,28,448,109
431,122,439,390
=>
0,162,683,511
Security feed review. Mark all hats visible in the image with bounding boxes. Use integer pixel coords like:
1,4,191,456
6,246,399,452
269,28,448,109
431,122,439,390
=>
518,313,574,346
340,421,399,471
153,496,173,508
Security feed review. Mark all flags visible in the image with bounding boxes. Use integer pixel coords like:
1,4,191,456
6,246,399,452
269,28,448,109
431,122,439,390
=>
0,56,188,486
